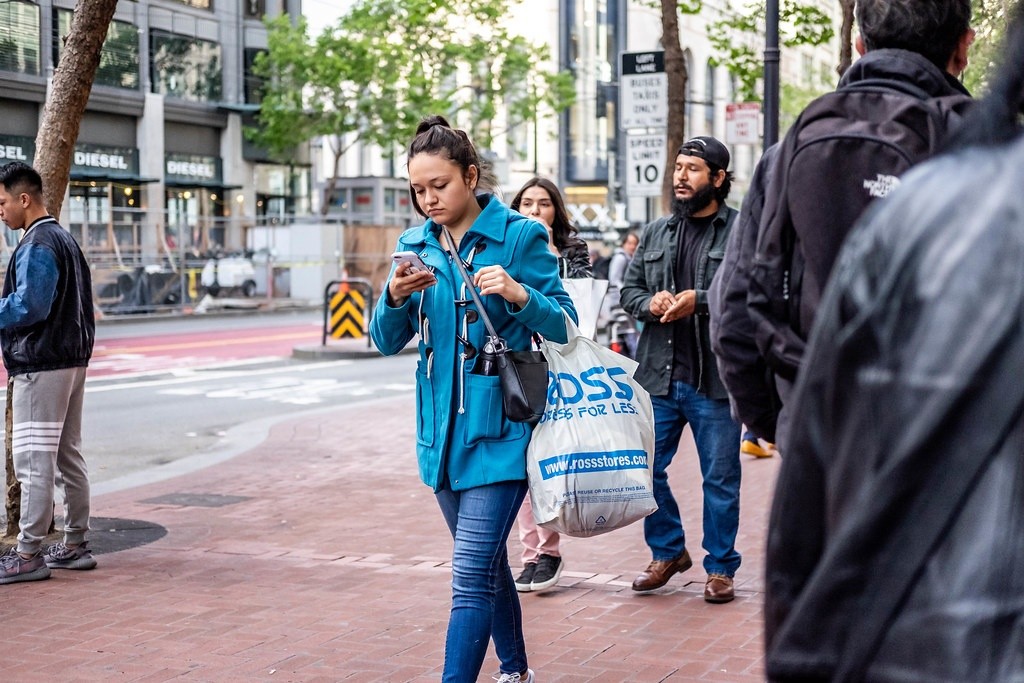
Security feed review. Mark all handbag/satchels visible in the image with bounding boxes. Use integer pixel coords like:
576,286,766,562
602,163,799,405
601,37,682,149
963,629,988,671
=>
526,307,658,537
494,346,551,422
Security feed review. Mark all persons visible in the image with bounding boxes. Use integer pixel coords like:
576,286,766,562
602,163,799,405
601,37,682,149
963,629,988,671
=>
606,233,640,357
511,178,596,592
709,0,984,445
619,136,742,604
741,429,778,457
369,116,579,683
0,160,98,583
764,0,1024,683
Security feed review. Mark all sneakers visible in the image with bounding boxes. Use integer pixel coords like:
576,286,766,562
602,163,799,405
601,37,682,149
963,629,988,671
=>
43,540,97,569
0,547,52,584
492,668,535,683
529,554,564,590
514,562,538,592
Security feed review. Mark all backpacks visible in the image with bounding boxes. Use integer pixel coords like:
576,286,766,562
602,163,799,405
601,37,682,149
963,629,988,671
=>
747,87,972,384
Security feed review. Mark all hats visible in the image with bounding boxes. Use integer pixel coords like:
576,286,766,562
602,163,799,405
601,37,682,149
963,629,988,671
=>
678,136,729,171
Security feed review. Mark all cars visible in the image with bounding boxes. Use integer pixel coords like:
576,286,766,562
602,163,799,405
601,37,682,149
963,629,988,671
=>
93,244,292,317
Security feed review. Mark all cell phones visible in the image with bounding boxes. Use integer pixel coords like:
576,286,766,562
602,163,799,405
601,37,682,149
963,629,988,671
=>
390,250,437,283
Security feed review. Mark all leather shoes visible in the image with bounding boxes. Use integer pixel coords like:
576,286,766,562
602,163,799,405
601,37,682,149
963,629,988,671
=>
704,574,735,602
632,547,692,591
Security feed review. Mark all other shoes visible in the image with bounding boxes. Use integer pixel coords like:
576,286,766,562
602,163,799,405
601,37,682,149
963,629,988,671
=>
739,440,773,457
767,441,778,449
